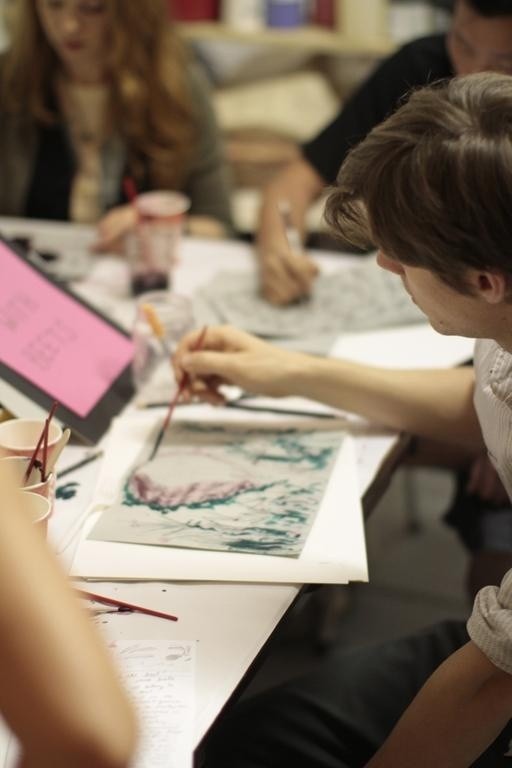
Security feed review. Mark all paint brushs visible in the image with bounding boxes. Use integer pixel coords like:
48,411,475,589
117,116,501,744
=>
78,592,178,621
26,401,58,484
148,323,210,462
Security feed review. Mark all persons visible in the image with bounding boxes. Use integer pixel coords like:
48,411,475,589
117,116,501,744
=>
172,70,511,768
256,0,512,309
0,451,142,768
1,0,239,258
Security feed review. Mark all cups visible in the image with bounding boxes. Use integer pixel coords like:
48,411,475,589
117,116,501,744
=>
0,416,64,544
134,190,192,268
129,228,173,297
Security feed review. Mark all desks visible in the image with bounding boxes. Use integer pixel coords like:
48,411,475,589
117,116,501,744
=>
0,217,484,768
